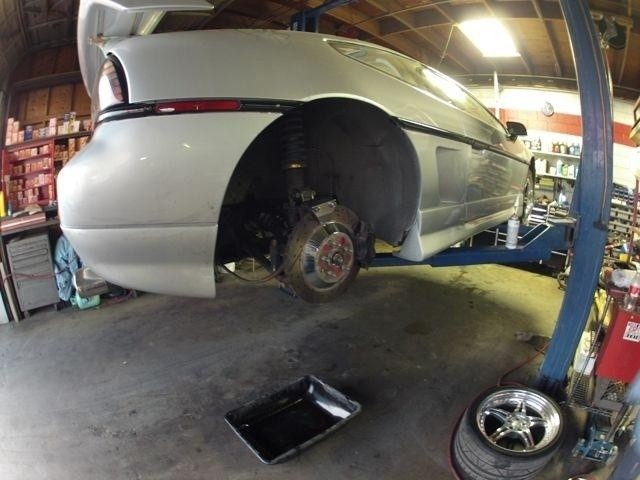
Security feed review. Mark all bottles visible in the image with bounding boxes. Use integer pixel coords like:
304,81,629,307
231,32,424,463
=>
536,136,541,150
505,212,520,250
624,271,640,312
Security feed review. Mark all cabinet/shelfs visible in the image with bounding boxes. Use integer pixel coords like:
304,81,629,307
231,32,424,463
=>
9,235,61,319
1,133,94,214
527,147,581,191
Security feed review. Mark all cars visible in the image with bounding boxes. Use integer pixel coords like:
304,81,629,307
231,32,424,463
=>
56,1,537,300
